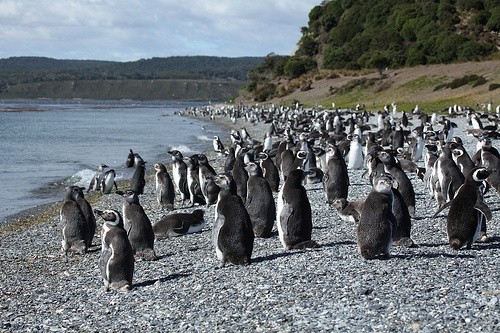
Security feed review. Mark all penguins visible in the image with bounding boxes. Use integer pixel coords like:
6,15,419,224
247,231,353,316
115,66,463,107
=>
73,191,96,254
94,209,135,292
124,149,138,168
149,100,500,250
86,164,109,195
101,169,118,194
357,176,399,264
133,153,147,170
130,161,148,194
59,186,90,263
114,190,158,262
206,173,255,270
